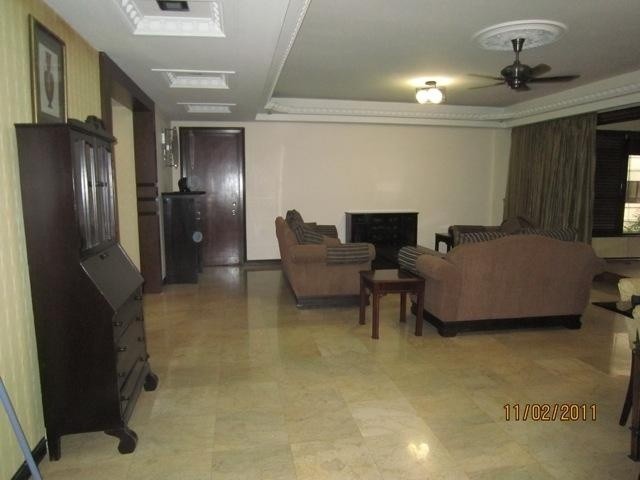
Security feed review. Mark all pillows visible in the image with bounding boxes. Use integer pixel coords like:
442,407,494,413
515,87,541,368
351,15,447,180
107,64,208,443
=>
283,207,328,245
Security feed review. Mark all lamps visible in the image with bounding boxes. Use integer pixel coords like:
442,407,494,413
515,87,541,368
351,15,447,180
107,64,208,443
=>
415,80,446,105
500,53,534,90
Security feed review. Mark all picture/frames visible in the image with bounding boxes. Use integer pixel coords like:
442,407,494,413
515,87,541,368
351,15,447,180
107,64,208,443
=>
28,13,70,124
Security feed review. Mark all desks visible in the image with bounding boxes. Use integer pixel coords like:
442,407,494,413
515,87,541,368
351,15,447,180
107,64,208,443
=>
359,268,426,340
435,231,453,253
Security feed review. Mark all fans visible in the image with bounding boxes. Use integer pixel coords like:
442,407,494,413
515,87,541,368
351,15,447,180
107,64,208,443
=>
466,38,582,92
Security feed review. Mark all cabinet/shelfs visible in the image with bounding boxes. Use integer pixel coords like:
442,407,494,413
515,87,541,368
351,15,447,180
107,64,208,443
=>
13,114,159,462
161,188,209,285
344,212,420,270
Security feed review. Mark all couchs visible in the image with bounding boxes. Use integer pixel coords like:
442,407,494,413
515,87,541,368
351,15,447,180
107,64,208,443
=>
397,233,608,339
274,216,376,310
447,215,579,248
